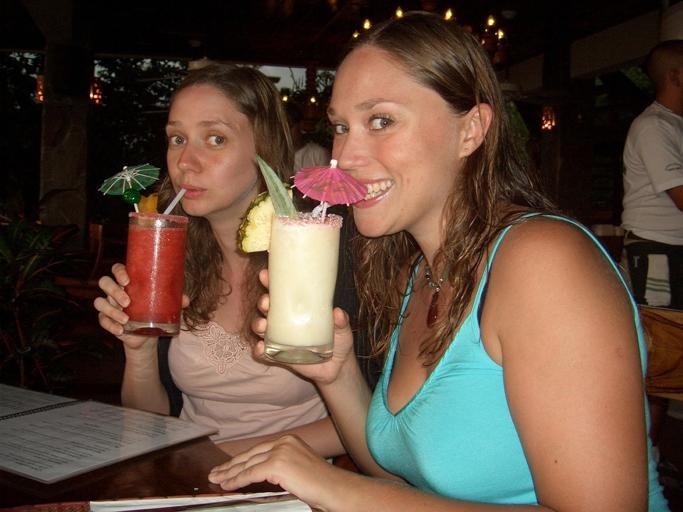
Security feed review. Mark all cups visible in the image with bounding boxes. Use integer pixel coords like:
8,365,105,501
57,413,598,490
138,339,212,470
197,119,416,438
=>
121,209,190,337
264,211,345,368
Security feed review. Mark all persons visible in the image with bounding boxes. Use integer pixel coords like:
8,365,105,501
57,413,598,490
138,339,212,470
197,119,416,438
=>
621,38,683,307
209,11,671,512
92,62,346,464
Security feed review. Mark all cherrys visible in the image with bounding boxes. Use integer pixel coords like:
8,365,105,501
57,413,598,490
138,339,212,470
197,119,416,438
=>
123,188,141,205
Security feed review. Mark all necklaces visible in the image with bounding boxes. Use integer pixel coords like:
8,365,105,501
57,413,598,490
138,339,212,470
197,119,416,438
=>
424,263,450,329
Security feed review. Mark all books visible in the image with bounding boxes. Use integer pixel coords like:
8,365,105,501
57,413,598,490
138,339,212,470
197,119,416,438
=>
0,384,218,484
